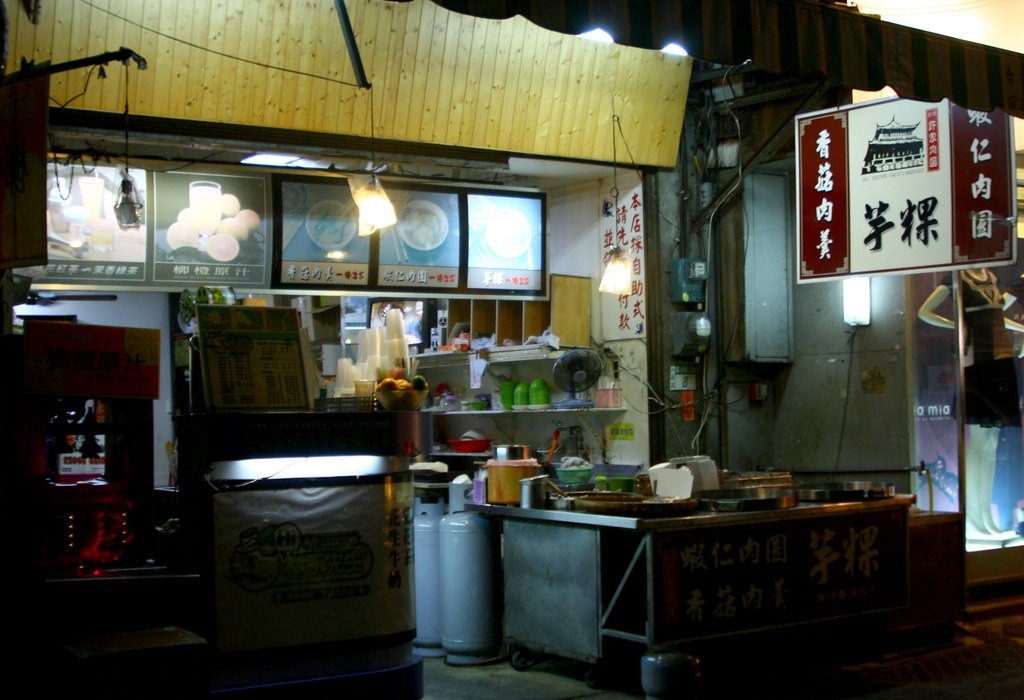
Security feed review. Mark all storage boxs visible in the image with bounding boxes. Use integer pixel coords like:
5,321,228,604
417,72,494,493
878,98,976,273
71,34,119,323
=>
595,389,622,408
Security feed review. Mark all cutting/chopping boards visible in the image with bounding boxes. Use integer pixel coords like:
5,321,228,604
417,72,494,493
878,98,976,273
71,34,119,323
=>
574,495,699,515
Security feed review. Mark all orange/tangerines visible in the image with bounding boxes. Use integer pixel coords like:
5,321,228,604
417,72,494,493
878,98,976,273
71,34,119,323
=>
376,376,413,391
168,195,260,262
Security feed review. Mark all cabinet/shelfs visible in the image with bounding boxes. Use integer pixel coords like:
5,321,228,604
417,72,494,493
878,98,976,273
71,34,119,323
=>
409,344,628,456
446,274,590,348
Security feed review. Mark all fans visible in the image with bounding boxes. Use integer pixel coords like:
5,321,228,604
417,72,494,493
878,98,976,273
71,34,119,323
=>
551,349,602,409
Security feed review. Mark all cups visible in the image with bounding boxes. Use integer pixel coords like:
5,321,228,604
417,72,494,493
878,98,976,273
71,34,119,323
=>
336,307,410,391
521,483,545,509
353,380,375,411
112,222,145,261
188,180,222,235
78,176,104,213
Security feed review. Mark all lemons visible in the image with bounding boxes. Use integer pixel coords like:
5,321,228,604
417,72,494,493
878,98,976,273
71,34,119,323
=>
412,375,426,391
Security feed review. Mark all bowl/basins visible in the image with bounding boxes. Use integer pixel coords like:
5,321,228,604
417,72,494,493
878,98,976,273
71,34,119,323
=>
498,381,513,410
492,444,532,460
518,473,549,485
398,199,449,252
460,430,486,439
593,388,623,409
514,383,529,405
305,199,357,251
528,378,550,404
447,438,493,453
555,467,593,484
469,401,488,411
486,209,533,258
609,477,634,494
377,390,430,411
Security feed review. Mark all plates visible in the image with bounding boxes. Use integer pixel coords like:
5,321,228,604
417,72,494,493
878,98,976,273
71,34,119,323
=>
528,404,550,410
510,405,528,410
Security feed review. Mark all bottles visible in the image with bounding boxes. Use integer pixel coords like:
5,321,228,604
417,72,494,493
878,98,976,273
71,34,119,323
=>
47,177,73,234
